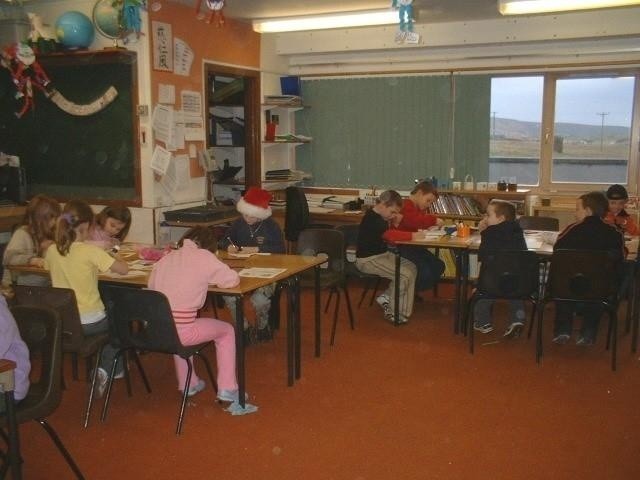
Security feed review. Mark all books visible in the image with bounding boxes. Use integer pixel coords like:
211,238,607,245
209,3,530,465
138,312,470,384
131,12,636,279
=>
274,132,314,144
264,168,315,182
426,194,481,216
265,94,304,106
413,223,457,243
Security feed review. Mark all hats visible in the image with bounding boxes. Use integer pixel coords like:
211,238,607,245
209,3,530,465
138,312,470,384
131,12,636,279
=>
236,187,273,220
606,184,628,200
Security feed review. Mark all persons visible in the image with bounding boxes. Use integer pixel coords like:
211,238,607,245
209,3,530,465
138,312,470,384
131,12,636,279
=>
356,190,419,325
602,183,640,236
0,192,75,351
44,196,128,397
215,186,284,342
148,224,250,402
470,200,530,337
85,204,132,251
0,260,31,408
389,180,446,300
549,192,624,346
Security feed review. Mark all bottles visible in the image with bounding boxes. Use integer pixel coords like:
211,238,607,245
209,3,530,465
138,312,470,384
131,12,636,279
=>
508,175,518,191
463,175,474,190
497,177,506,191
459,220,464,228
111,245,121,253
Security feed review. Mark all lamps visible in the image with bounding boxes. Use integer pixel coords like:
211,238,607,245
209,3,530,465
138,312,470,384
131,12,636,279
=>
497,1,640,18
250,5,418,38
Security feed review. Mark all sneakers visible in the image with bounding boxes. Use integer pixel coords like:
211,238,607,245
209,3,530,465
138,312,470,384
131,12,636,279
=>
552,333,571,346
576,338,593,346
473,321,494,334
216,390,249,402
93,368,108,399
376,294,408,324
504,322,524,338
183,381,205,396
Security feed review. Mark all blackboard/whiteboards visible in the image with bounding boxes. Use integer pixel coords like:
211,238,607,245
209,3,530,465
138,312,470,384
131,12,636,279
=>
0,48,143,208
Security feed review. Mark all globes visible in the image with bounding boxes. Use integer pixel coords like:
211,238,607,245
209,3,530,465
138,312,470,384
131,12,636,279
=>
92,0,135,50
55,11,95,51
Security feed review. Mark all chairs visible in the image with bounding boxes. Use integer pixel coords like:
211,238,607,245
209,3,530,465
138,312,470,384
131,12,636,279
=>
539,247,625,372
7,302,86,479
11,285,133,428
324,226,391,329
98,286,224,438
461,250,540,366
517,217,560,306
270,228,355,347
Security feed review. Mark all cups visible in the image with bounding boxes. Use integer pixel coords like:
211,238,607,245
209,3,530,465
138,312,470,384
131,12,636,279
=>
265,122,276,140
487,183,497,191
452,181,462,191
476,182,487,191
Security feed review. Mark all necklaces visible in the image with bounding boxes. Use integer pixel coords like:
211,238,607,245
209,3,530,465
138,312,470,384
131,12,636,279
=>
244,219,267,239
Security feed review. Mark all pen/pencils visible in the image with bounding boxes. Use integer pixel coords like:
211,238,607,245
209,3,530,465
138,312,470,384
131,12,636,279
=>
226,237,240,253
366,184,377,207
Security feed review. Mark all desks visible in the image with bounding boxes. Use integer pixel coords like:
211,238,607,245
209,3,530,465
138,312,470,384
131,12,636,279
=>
0,357,21,480
393,223,640,354
9,236,327,407
533,200,638,277
158,193,413,286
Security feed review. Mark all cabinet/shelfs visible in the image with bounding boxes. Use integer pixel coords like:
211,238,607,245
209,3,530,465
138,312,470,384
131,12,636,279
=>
262,94,314,192
421,186,531,285
